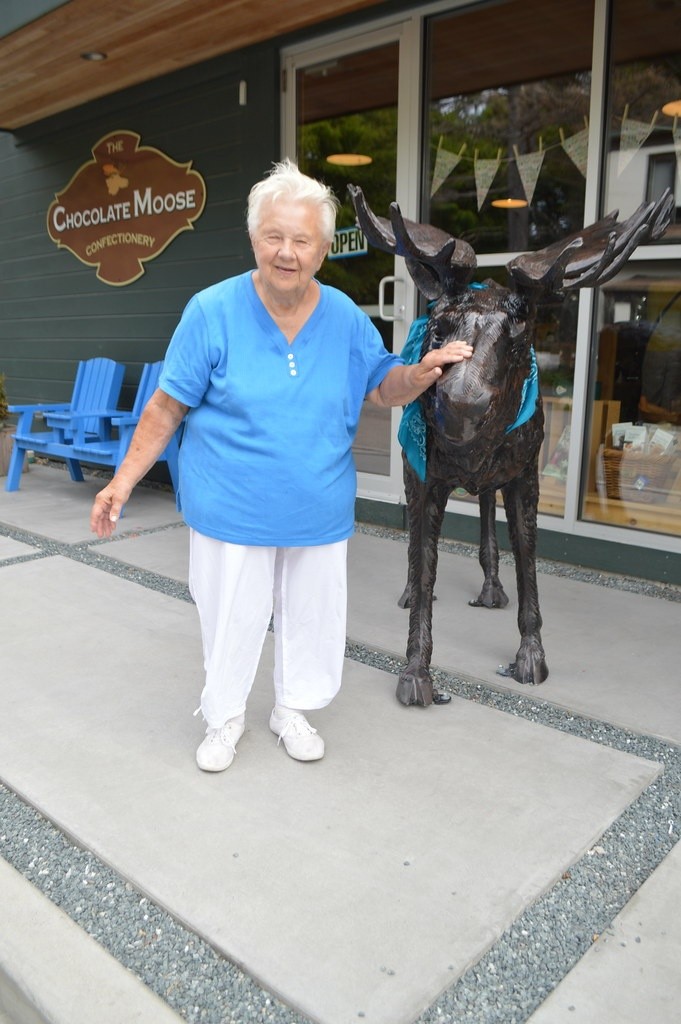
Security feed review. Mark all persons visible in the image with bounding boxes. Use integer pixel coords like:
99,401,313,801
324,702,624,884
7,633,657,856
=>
89,156,474,772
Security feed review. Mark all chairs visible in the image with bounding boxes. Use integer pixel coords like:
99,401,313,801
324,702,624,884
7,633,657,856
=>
4,356,188,519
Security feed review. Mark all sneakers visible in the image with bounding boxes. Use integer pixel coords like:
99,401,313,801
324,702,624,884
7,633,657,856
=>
269,706,325,761
196,722,246,771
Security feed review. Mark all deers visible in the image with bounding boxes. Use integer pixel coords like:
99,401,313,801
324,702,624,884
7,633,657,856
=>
347,182,677,707
